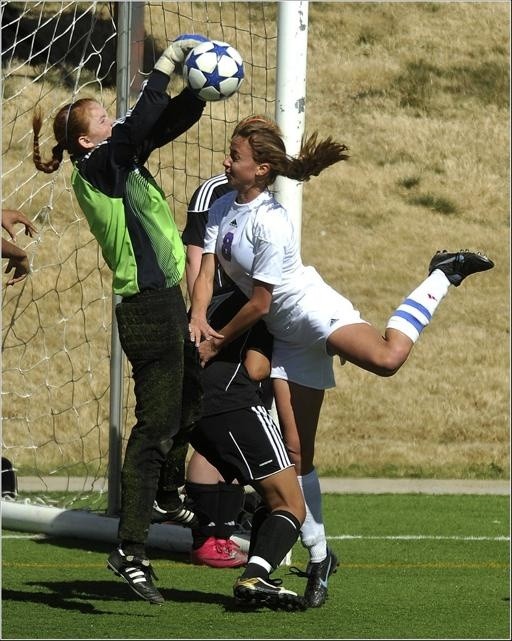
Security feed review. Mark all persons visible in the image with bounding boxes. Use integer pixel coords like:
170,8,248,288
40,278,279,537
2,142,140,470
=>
187,121,494,608
33,34,245,607
178,114,284,568
2,210,39,287
184,284,309,613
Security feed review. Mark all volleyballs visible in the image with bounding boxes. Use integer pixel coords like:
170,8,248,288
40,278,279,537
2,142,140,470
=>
179,38,246,102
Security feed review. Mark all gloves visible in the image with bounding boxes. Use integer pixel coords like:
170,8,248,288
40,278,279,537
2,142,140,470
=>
154,35,209,77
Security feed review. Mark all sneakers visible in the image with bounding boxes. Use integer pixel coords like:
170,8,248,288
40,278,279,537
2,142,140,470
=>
427,250,495,287
105,543,166,607
303,547,341,608
151,499,196,525
232,577,307,612
189,539,249,567
